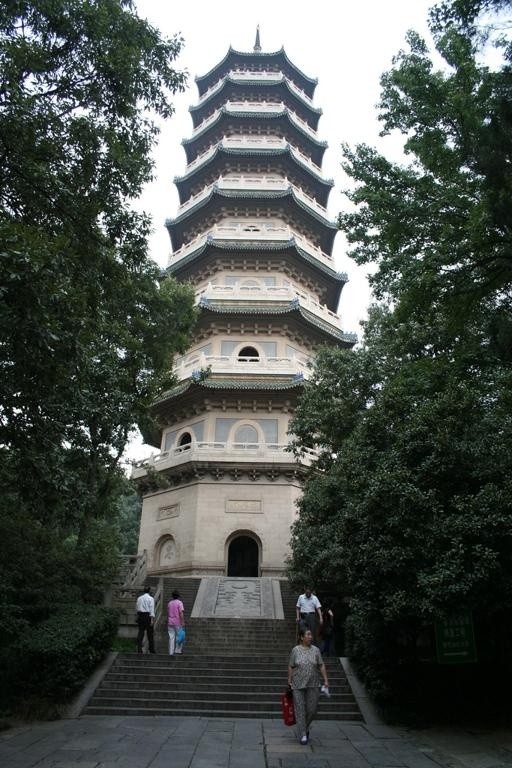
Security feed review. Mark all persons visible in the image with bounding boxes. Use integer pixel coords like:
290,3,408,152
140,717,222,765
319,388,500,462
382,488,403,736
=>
166,590,187,656
321,599,335,657
135,586,156,655
288,627,330,746
296,587,323,647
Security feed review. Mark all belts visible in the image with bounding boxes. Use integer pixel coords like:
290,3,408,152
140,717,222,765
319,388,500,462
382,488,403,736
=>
301,612,315,614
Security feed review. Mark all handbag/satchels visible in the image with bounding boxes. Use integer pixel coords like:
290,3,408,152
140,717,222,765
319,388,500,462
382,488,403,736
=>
176,626,186,643
324,625,334,637
282,685,295,726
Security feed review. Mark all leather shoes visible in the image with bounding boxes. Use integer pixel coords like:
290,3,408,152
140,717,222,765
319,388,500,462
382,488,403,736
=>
300,732,309,745
